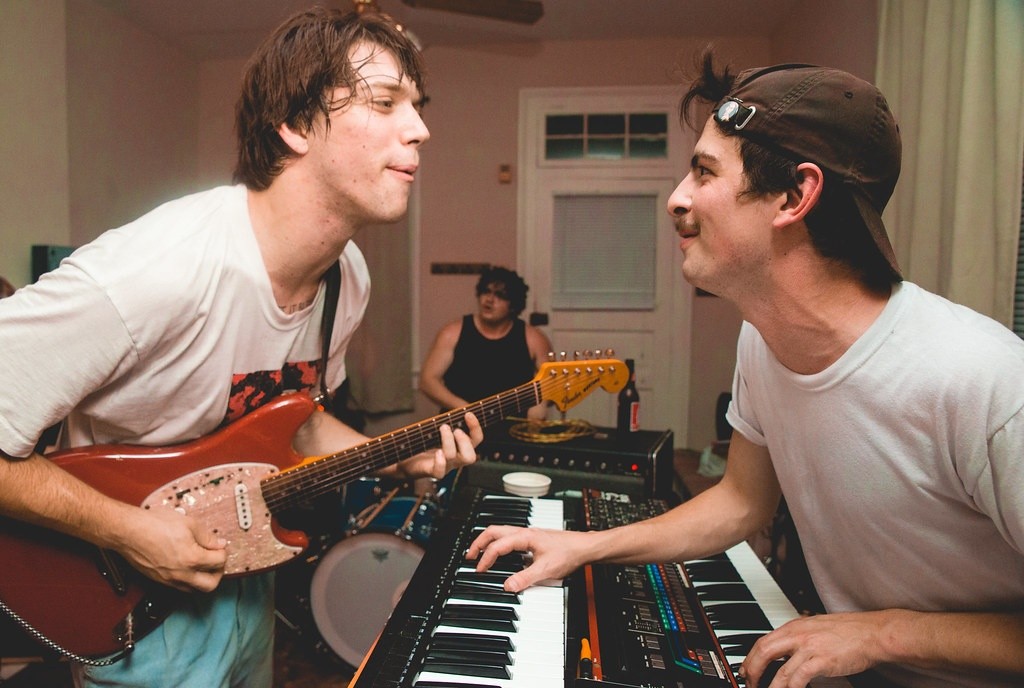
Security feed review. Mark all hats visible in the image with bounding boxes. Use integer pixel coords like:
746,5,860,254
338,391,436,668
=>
711,63,903,281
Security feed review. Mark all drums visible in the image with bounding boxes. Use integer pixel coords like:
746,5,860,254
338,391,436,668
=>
309,495,443,670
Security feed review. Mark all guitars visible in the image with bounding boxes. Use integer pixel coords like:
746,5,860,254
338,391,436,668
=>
0,348,630,659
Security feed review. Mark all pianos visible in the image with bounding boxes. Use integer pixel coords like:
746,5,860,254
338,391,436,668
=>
353,484,853,688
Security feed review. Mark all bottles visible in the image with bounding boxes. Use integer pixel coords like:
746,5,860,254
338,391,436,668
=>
617,358,639,433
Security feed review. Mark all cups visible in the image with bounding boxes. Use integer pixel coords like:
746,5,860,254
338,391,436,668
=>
502,472,551,497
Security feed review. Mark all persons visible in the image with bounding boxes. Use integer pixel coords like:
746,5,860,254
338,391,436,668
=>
0,0,486,688
463,39,1024,688
418,265,557,424
